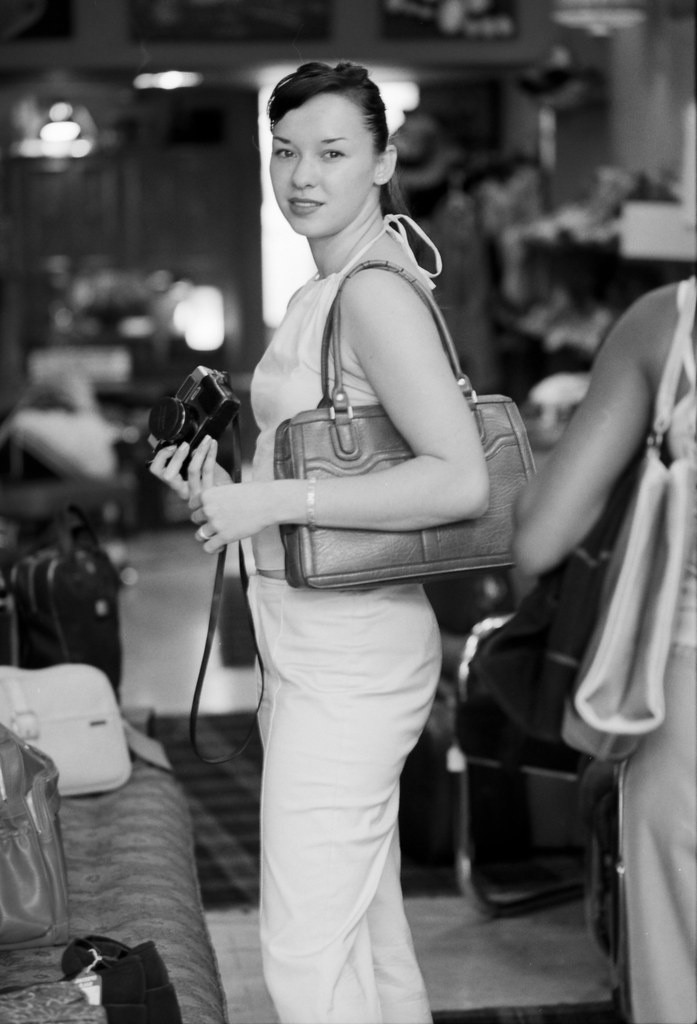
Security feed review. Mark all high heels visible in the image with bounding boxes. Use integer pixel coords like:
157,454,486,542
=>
0,932,183,1024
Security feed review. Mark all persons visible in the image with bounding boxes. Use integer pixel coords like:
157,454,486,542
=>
510,276,697,1024
150,62,492,1024
392,117,492,393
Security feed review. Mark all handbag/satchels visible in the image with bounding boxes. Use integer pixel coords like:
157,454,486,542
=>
0,726,71,952
562,279,697,760
278,263,541,592
0,664,172,797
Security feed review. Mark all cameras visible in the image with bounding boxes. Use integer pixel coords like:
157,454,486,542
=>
148,365,241,482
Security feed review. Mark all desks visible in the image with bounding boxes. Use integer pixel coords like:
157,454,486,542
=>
0,767,229,1024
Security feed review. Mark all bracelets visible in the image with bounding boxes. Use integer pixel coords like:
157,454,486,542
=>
308,478,316,531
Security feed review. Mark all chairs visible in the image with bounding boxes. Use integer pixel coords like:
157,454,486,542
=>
452,468,641,959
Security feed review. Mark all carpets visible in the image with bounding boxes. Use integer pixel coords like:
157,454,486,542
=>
147,708,455,909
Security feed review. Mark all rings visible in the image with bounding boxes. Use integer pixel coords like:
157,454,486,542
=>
199,527,210,540
